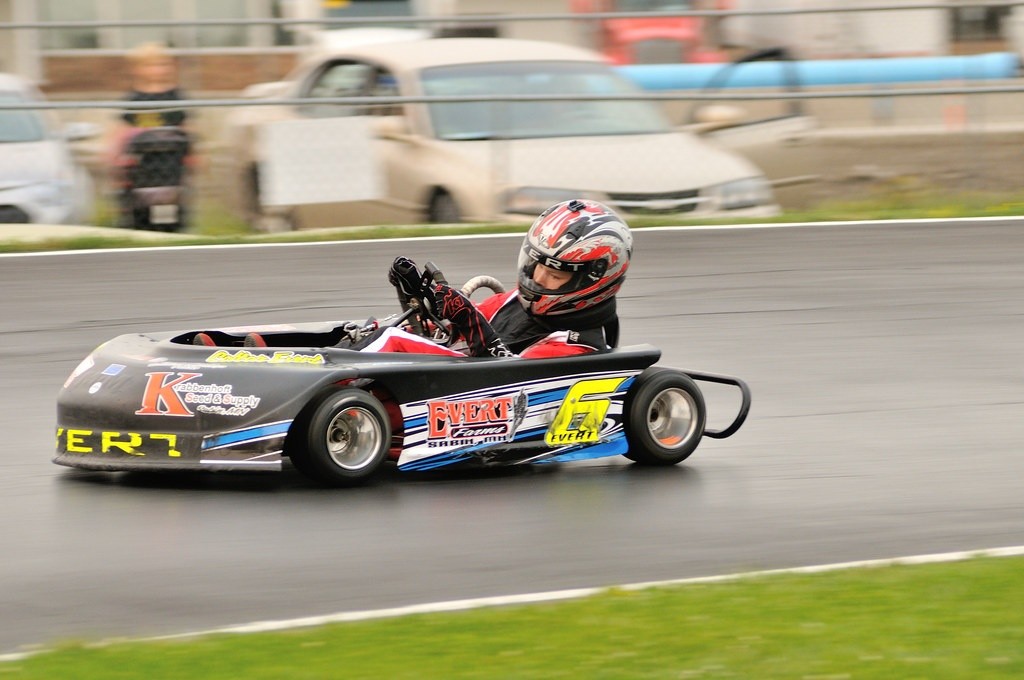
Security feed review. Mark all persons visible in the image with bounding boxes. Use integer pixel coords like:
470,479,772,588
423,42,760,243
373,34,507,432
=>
193,208,618,365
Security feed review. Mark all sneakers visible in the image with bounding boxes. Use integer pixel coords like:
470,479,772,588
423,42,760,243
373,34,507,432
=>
244,332,266,347
193,333,216,346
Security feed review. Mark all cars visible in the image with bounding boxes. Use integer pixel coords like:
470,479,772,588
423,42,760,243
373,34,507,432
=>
0,72,93,232
233,26,829,251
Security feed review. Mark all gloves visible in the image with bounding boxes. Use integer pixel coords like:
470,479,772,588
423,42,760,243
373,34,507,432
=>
435,282,492,351
387,256,426,305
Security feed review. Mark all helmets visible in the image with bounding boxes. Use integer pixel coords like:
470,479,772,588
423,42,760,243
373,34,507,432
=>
515,198,634,316
